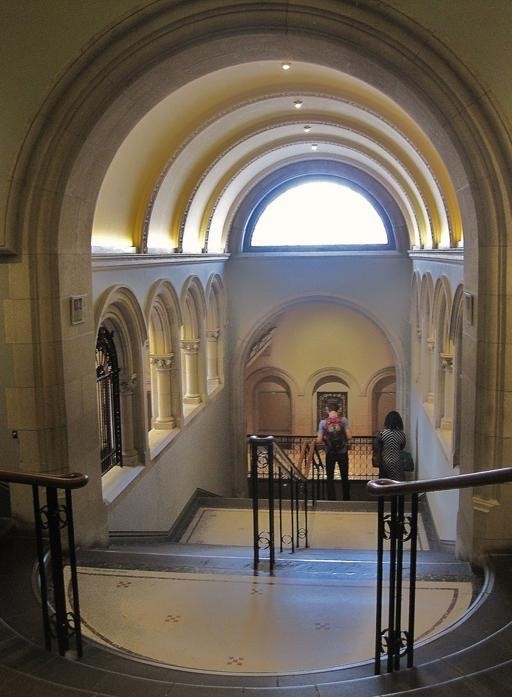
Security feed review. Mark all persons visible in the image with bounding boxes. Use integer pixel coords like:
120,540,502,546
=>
317,403,353,501
377,411,406,482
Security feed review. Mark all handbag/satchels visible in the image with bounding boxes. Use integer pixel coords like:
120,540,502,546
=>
400,451,414,472
372,433,383,468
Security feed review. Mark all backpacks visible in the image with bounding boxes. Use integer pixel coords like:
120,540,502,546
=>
322,417,346,452
